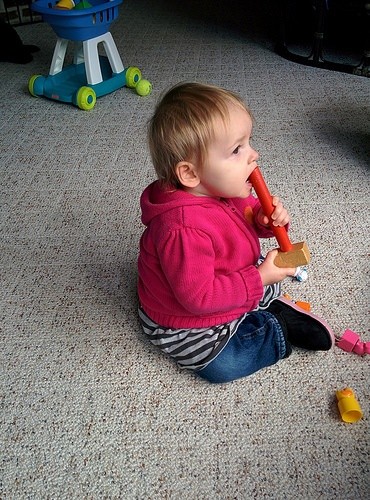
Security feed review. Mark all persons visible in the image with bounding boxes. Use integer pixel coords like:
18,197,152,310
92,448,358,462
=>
137,83,335,383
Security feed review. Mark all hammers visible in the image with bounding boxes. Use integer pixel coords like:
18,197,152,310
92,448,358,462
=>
248,167,311,269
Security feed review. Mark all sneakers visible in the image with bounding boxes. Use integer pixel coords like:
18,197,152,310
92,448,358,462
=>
265,296,334,351
